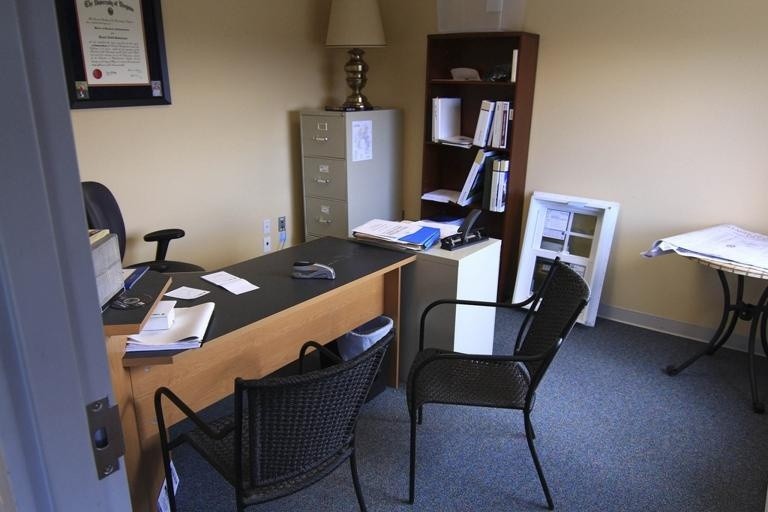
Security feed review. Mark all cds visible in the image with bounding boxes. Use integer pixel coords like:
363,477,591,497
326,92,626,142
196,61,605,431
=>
111,293,152,309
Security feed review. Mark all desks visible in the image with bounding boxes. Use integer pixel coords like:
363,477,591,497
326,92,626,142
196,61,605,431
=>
101,235,416,511
350,227,503,387
640,224,767,413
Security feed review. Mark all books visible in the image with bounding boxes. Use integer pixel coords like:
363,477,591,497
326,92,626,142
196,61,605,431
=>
88,227,111,243
421,147,509,212
122,265,150,291
429,93,511,150
510,46,519,83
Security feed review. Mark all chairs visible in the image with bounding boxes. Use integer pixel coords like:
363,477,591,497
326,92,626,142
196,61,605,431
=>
407,254,591,507
82,182,203,271
153,333,395,511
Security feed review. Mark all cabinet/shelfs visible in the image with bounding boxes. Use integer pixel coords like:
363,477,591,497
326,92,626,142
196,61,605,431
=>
299,108,402,239
422,30,540,305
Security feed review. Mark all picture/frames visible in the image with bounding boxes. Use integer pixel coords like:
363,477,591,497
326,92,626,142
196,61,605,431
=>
56,1,172,110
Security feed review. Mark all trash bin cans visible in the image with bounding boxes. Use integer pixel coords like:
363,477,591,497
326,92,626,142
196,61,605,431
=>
319,314,394,381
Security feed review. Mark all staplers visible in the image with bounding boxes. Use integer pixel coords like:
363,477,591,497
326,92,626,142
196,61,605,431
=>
291,261,335,279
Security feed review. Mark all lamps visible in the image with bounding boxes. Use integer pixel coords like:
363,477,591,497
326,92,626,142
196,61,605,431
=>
326,0,388,111
